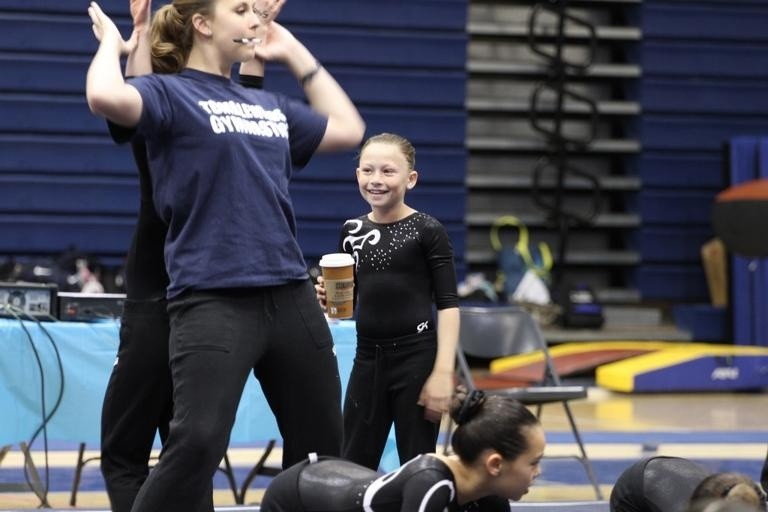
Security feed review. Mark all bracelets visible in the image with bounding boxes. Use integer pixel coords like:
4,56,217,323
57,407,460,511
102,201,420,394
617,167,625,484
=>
298,61,320,89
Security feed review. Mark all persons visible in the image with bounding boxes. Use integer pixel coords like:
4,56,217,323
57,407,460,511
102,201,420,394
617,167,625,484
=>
608,456,768,512
83,1,366,512
100,1,287,512
257,385,547,512
315,133,459,469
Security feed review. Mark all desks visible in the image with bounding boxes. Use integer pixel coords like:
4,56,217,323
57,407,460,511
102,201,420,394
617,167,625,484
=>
0,319,401,512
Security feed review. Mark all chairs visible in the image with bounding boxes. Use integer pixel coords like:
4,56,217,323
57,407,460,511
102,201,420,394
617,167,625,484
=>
444,306,604,502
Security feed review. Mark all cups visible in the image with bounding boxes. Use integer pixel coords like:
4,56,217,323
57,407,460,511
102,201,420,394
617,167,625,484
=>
317,251,356,321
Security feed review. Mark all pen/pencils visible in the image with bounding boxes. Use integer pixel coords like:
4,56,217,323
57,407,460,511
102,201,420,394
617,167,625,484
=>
234,38,262,44
